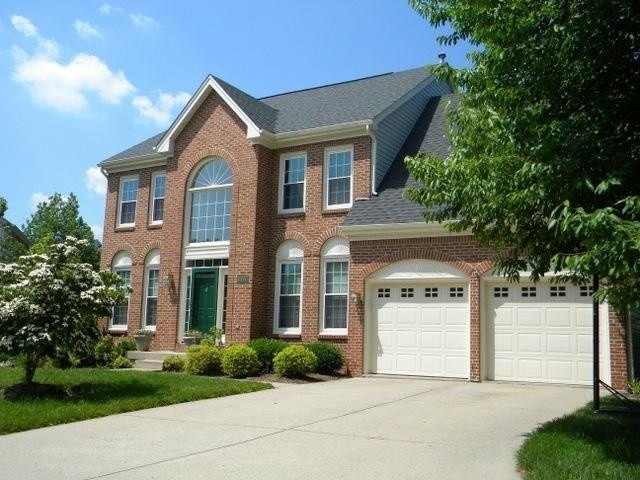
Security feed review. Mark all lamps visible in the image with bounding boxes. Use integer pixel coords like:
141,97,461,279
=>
351,292,362,309
163,270,172,287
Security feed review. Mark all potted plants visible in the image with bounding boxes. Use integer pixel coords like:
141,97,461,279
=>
133,328,152,351
182,330,200,346
